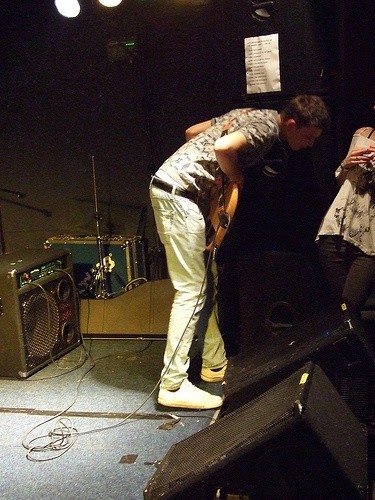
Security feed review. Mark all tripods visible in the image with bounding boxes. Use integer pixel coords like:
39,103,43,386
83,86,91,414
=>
84,156,127,299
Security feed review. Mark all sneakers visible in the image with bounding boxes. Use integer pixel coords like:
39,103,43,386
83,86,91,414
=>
200,365,227,382
158,378,223,409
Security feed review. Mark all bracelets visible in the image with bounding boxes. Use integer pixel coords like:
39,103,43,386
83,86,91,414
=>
340,160,351,171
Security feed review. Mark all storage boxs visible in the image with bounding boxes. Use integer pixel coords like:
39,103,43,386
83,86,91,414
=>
44,235,146,300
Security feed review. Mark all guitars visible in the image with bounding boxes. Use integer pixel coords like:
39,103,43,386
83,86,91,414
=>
207,181,240,251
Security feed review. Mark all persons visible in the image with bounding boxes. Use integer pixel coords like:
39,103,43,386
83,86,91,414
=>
149,94,328,410
315,127,375,322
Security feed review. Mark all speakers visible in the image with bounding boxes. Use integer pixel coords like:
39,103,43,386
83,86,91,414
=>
222,296,375,424
143,361,368,500
0,247,80,379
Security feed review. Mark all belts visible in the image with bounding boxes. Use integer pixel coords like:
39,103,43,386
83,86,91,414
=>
150,176,198,204
